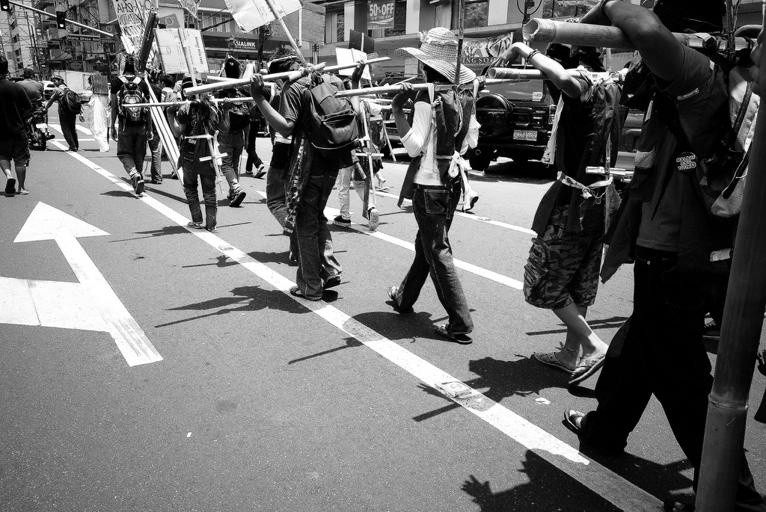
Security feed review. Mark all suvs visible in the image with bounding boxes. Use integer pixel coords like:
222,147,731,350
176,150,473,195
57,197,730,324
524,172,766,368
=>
366,74,424,161
468,49,632,171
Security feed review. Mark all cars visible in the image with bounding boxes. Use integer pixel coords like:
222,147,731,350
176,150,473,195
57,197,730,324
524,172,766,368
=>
37,80,55,98
618,111,646,154
72,88,93,104
734,24,763,40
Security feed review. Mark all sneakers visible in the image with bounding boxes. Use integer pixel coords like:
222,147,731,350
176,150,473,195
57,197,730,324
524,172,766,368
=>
130,164,266,233
288,206,378,301
462,193,478,211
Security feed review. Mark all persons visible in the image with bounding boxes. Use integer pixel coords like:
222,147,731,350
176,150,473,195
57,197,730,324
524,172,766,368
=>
40,74,81,152
387,27,479,343
0,56,45,197
107,48,379,303
495,2,766,512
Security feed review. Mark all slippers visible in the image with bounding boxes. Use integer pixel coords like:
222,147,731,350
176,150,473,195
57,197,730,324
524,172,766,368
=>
569,357,604,384
18,188,30,195
435,324,473,344
5,179,17,194
387,286,413,314
535,352,574,373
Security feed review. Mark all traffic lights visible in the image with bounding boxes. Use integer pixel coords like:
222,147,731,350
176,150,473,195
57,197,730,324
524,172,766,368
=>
0,0,9,11
56,11,65,28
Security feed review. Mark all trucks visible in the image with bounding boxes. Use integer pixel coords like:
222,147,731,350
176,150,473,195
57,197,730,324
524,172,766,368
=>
50,70,111,93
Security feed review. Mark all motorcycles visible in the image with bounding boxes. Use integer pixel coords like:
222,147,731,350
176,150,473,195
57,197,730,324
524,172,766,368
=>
25,105,54,152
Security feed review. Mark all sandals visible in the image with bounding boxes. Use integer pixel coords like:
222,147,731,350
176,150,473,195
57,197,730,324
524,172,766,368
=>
564,408,586,432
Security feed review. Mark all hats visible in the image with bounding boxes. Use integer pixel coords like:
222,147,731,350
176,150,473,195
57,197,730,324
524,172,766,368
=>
394,27,476,84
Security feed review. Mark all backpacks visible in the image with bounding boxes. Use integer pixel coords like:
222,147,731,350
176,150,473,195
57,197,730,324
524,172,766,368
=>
116,76,150,127
56,88,81,115
295,71,359,157
